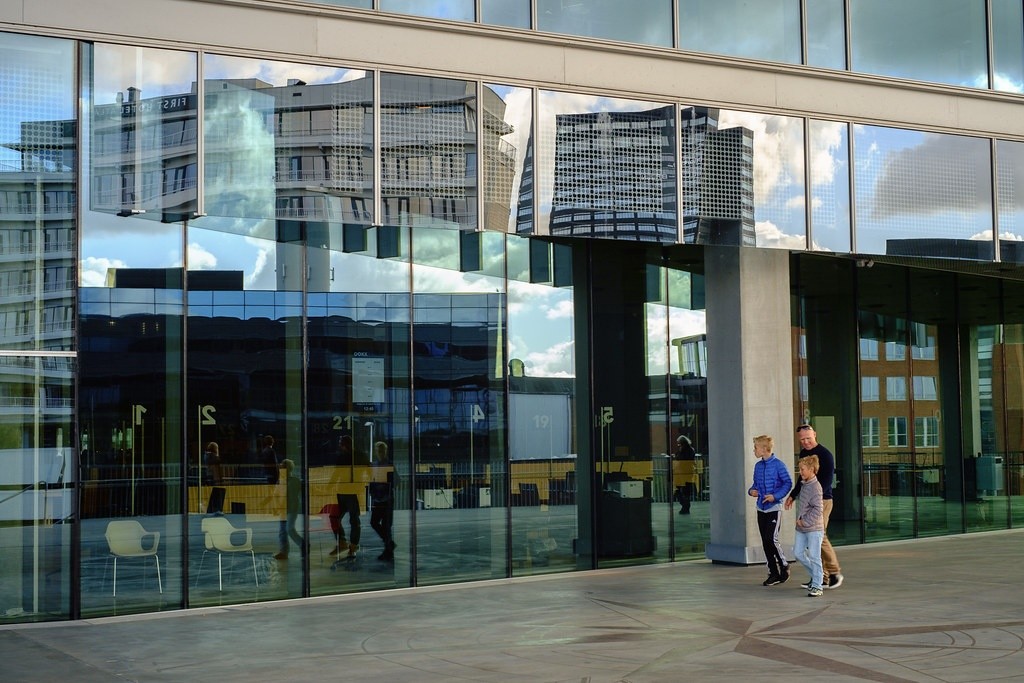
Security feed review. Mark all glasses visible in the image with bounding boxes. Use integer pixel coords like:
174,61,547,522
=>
797,426,813,432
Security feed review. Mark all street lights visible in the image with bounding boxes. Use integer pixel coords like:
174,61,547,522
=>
364,421,374,512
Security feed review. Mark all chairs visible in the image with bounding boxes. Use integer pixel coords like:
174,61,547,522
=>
101,520,162,597
194,517,259,592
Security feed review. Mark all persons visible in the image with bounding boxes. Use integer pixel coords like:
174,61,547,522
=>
785,424,845,589
368,442,397,560
676,435,697,515
748,435,792,587
203,434,310,561
792,455,825,597
323,436,372,560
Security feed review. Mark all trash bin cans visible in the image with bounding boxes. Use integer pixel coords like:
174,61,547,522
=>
975,454,1003,496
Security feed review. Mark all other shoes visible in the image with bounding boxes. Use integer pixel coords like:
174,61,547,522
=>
679,506,690,515
763,574,780,586
378,541,398,561
779,564,790,583
347,550,358,562
301,544,310,558
273,551,288,560
329,543,350,556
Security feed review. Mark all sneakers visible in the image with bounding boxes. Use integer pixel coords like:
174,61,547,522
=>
801,577,829,590
828,574,844,590
808,587,824,597
